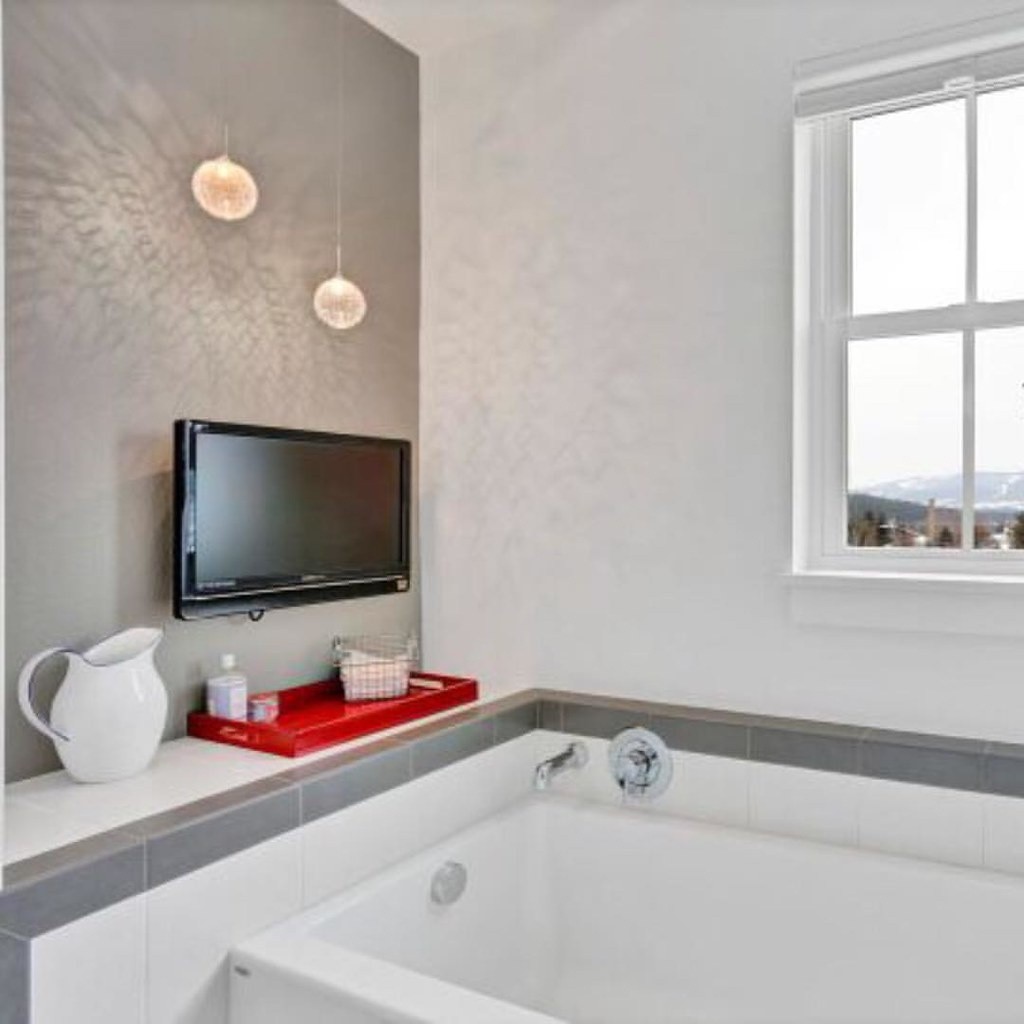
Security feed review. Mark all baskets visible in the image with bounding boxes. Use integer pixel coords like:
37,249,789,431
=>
331,631,417,702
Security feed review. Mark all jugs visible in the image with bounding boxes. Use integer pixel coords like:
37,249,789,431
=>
17,627,168,782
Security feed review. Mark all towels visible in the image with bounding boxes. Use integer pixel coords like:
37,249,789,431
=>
340,648,410,700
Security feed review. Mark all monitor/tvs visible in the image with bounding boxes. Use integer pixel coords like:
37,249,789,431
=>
169,417,412,621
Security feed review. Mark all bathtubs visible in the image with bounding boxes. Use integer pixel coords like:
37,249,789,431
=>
232,799,1024,1022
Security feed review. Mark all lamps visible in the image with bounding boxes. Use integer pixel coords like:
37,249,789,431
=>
193,114,265,224
313,2,369,331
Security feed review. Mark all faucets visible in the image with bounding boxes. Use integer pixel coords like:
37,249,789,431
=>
530,738,585,796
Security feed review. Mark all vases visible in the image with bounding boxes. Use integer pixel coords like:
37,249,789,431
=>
18,624,169,789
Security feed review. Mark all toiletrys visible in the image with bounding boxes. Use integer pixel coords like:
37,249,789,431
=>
206,652,249,723
